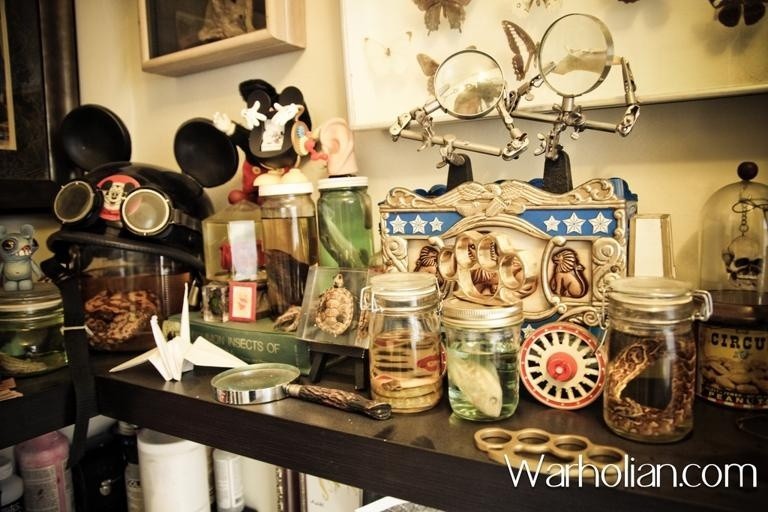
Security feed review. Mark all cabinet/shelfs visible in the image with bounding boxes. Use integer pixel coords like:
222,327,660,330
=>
134,0,307,78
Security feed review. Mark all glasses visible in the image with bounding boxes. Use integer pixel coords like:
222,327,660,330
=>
50,178,186,238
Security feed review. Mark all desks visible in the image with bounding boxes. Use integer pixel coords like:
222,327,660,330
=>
0,350,768,512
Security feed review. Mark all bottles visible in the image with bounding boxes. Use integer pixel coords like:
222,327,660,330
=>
364,272,768,445
258,176,373,328
0,420,246,512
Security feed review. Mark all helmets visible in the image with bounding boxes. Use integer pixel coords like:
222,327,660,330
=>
46,158,216,273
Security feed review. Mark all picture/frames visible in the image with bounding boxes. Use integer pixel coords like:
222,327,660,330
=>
0,0,84,221
228,281,257,323
